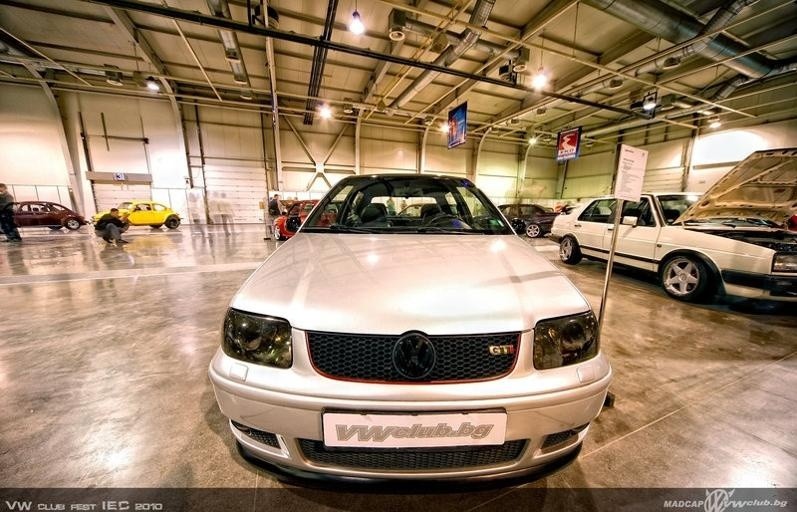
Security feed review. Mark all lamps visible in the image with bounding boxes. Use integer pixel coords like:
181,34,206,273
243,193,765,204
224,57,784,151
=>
642,90,658,110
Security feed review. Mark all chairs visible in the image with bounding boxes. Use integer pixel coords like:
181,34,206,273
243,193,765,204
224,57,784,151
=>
421,204,446,227
360,202,388,229
596,206,613,218
665,209,680,222
625,209,644,225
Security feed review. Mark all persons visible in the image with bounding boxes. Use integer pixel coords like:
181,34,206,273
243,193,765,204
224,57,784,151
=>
264,194,297,235
189,192,236,238
95,208,128,243
0,183,22,242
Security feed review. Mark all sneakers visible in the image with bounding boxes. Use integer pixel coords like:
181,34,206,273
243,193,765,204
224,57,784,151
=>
103,237,127,243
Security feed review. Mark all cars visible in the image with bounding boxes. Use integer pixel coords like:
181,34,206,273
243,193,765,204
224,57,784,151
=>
13,201,86,230
207,173,612,494
496,203,558,239
89,200,183,229
270,199,345,240
548,147,796,308
